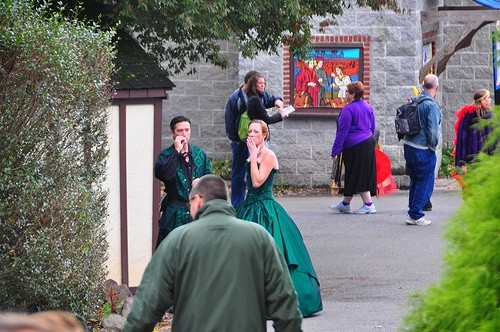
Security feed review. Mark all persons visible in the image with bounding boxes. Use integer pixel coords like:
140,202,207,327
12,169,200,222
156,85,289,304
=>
328,81,378,214
402,74,442,225
245,73,287,147
224,71,284,208
155,116,213,252
0,309,85,332
235,120,323,316
123,174,304,332
455,90,497,174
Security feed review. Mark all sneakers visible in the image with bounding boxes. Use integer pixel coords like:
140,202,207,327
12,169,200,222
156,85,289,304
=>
405,217,431,225
328,201,351,213
353,203,377,214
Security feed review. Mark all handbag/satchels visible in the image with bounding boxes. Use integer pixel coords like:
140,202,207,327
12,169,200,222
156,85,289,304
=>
375,148,397,196
238,111,250,141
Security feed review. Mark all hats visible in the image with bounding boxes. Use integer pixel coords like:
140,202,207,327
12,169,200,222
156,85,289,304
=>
474,89,488,102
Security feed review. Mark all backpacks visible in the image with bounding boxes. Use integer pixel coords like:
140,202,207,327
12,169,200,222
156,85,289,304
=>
395,96,439,142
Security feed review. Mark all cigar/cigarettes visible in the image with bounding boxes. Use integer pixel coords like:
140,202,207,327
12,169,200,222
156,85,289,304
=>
250,140,252,142
